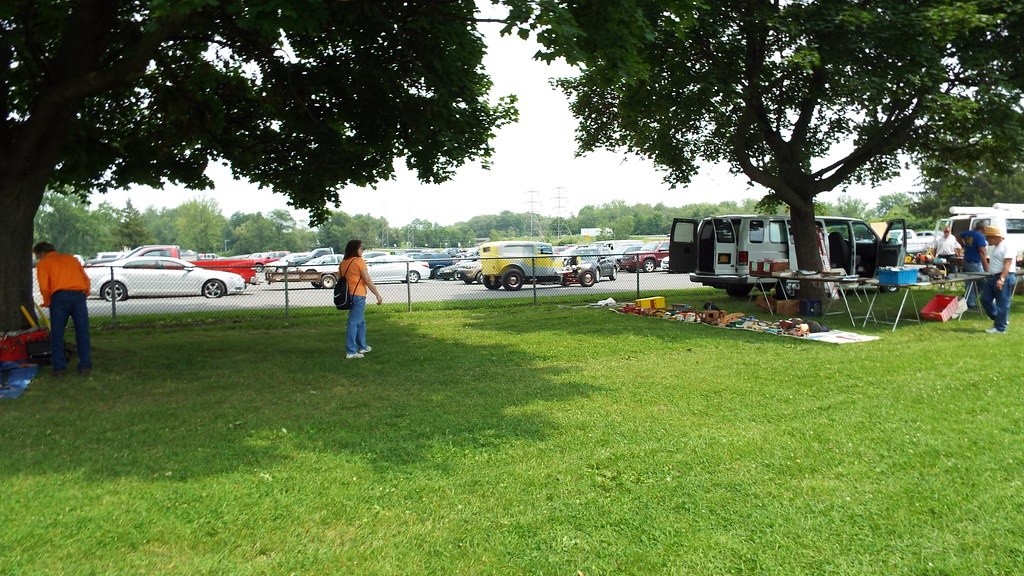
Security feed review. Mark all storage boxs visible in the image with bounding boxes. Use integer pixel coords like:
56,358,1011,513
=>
635,296,665,310
777,299,800,315
951,296,968,320
798,299,822,317
749,257,788,277
875,266,919,286
920,294,959,323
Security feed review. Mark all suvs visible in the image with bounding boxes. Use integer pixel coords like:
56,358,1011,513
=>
559,246,621,286
622,239,669,273
481,240,568,292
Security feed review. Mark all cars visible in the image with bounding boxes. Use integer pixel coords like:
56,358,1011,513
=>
320,255,431,285
82,256,247,299
247,247,347,273
366,238,644,282
887,227,936,255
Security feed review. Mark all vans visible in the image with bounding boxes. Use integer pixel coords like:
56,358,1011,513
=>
96,252,124,260
667,214,910,297
945,204,1024,264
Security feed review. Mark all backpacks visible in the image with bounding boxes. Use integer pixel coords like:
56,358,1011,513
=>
334,258,362,310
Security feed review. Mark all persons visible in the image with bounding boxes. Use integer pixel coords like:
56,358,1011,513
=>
934,225,962,274
955,220,989,310
981,225,1017,333
33,241,92,378
337,239,383,359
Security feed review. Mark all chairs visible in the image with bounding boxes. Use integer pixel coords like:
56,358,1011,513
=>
831,268,862,304
828,231,861,265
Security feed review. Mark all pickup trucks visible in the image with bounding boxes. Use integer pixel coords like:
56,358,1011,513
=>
94,246,263,289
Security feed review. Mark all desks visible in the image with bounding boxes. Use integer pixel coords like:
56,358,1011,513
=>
747,272,877,328
863,268,1024,332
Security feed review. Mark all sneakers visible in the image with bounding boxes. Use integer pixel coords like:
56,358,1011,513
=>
359,346,372,353
346,353,364,359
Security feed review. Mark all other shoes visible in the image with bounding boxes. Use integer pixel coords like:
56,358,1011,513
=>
985,326,1006,333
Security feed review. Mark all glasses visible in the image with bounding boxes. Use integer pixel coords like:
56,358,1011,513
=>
943,230,950,233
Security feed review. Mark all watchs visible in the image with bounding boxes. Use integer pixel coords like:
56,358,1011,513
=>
998,278,1005,281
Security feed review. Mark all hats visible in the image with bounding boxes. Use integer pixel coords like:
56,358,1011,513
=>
984,226,1002,237
